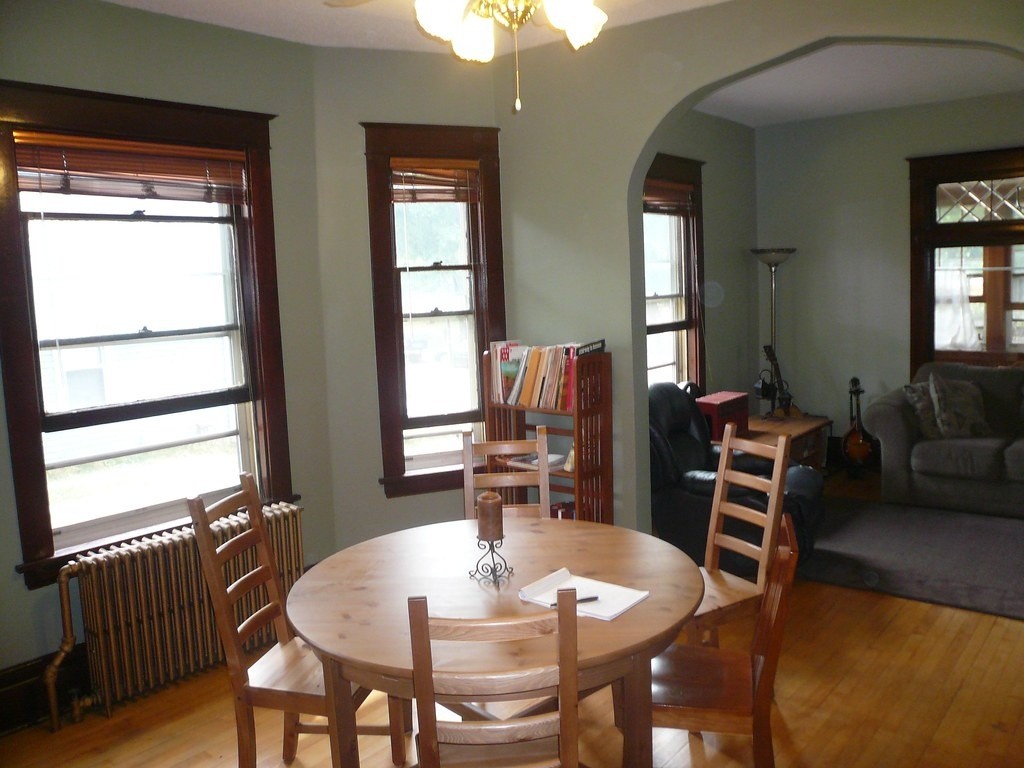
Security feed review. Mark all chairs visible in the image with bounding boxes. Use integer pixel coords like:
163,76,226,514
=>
189,474,408,767
677,423,792,647
611,510,803,768
407,588,581,766
461,424,551,521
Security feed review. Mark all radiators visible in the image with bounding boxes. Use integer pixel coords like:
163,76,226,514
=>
76,497,303,719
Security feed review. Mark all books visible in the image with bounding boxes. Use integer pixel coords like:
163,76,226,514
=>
505,449,564,471
486,334,607,415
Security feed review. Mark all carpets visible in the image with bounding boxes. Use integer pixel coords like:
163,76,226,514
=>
804,496,1024,620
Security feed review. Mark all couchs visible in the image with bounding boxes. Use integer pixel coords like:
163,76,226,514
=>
859,361,1024,517
647,381,822,578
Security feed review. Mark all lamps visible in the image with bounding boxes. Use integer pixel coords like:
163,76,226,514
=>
752,246,796,421
323,0,610,112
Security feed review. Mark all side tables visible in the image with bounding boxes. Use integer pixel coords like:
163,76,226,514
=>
708,411,833,478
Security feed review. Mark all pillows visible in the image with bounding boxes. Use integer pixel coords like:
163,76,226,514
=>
903,380,942,439
928,370,995,439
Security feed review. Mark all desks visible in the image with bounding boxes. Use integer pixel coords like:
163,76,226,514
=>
285,518,706,767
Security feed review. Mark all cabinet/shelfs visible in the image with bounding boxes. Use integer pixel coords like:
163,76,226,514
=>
481,351,613,527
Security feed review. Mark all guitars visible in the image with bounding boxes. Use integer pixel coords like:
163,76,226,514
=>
764,343,816,467
841,377,877,467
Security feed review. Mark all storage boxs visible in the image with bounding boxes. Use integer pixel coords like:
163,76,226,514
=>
694,391,749,440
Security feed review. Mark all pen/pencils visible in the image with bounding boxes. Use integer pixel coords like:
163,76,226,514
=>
550,597,598,607
